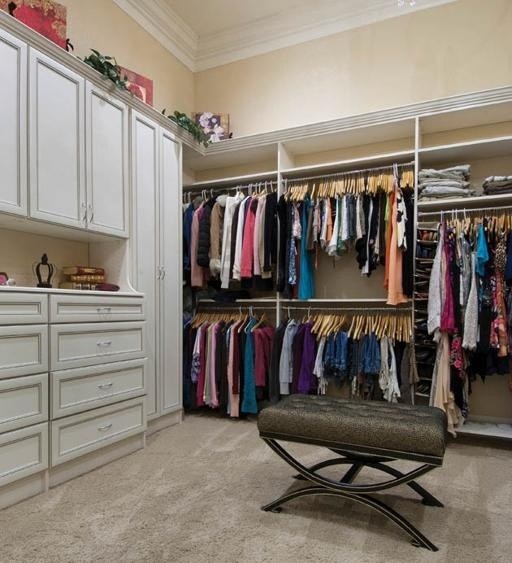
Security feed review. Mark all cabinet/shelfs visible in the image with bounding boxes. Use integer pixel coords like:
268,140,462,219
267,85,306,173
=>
0,11,28,232
89,96,183,438
49,288,147,489
181,96,512,441
0,285,49,512
29,27,131,243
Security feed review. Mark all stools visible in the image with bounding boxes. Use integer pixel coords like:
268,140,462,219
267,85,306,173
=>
257,393,448,552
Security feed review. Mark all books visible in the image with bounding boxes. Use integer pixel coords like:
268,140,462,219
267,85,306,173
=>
58,266,119,291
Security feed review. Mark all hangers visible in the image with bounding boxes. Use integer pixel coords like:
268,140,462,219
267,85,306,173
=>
434,203,512,239
279,159,414,201
190,302,413,344
